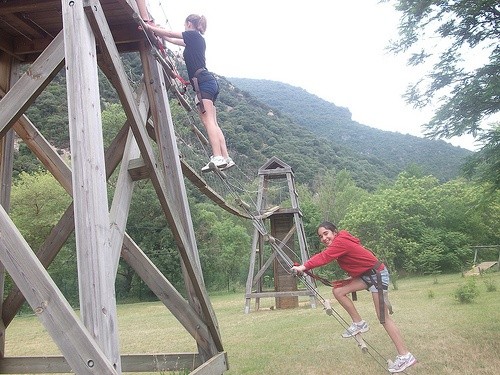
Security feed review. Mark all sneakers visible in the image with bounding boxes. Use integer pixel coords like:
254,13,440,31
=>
201,155,235,173
341,320,370,338
388,352,417,373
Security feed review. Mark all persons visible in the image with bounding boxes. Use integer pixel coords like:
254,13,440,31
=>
290,221,417,373
135,0,235,173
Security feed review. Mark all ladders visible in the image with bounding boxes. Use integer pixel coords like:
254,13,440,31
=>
132,12,409,375
